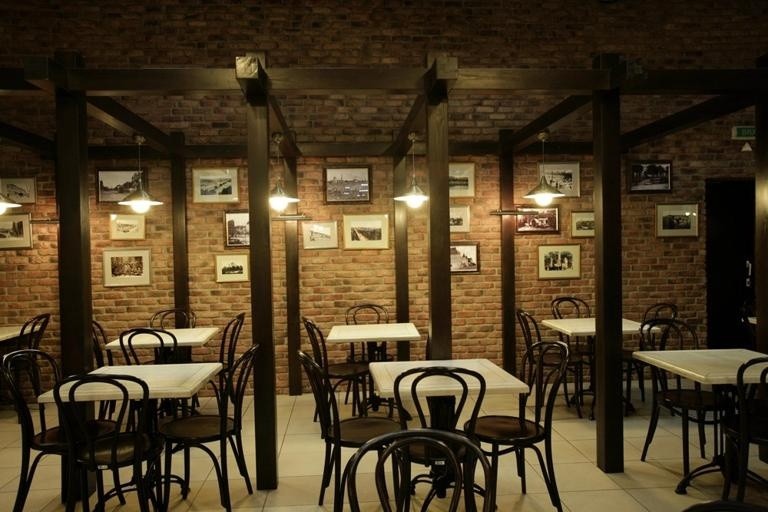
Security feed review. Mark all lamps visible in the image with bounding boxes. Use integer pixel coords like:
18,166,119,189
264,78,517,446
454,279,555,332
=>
269,133,300,212
521,127,565,206
394,130,430,210
118,134,164,214
